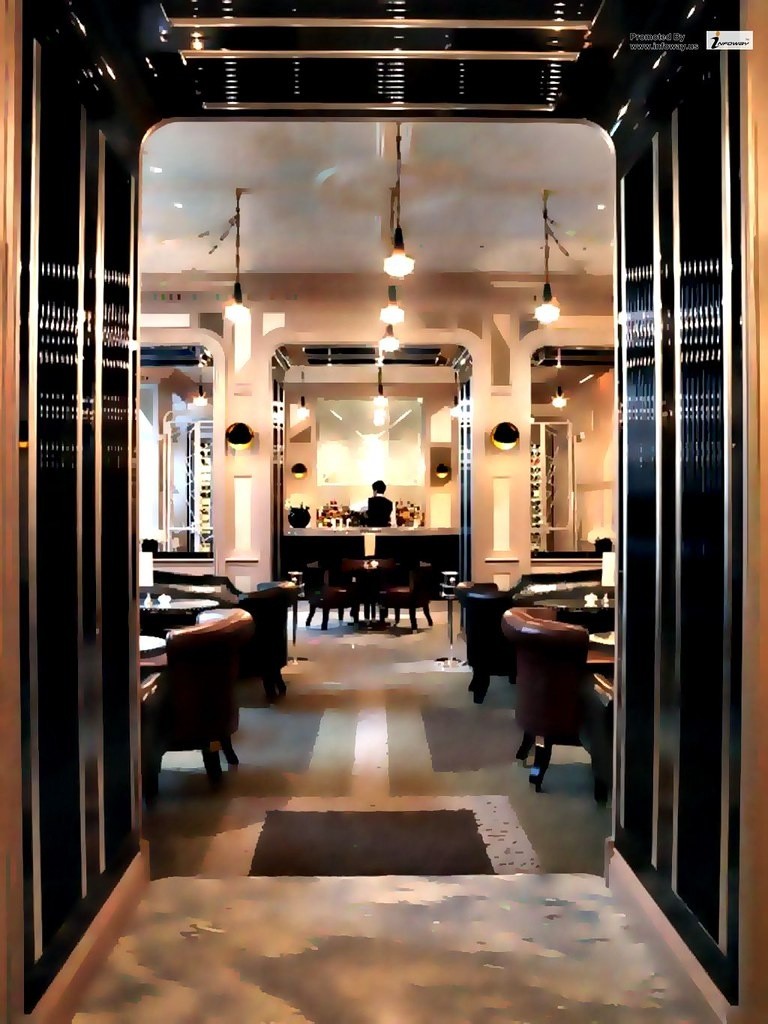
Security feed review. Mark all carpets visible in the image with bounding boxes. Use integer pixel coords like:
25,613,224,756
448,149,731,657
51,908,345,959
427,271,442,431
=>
248,810,498,881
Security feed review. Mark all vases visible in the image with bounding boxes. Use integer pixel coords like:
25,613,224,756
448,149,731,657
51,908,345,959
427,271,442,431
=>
289,508,310,529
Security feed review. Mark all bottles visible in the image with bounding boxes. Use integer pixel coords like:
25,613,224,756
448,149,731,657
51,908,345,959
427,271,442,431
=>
316,500,425,528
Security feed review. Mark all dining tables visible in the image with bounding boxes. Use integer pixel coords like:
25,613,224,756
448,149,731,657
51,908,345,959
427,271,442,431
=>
338,556,403,625
139,636,165,659
139,599,221,640
590,634,615,651
538,597,615,633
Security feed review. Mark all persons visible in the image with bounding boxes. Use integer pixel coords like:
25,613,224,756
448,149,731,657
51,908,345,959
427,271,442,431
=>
359,480,393,527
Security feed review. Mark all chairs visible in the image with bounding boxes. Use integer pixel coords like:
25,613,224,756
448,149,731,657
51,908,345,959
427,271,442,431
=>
137,609,252,787
456,583,518,705
209,581,297,702
380,564,434,633
591,674,614,807
140,673,166,797
513,569,605,593
305,563,352,631
503,606,615,795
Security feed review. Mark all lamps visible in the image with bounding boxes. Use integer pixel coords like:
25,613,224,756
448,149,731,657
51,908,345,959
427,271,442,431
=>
435,463,450,478
297,396,310,420
451,394,459,418
139,551,154,587
532,190,561,324
225,422,254,451
384,121,415,276
192,385,208,407
380,189,406,323
552,387,567,408
491,422,518,450
224,189,249,323
292,463,306,479
380,324,399,353
601,551,617,589
